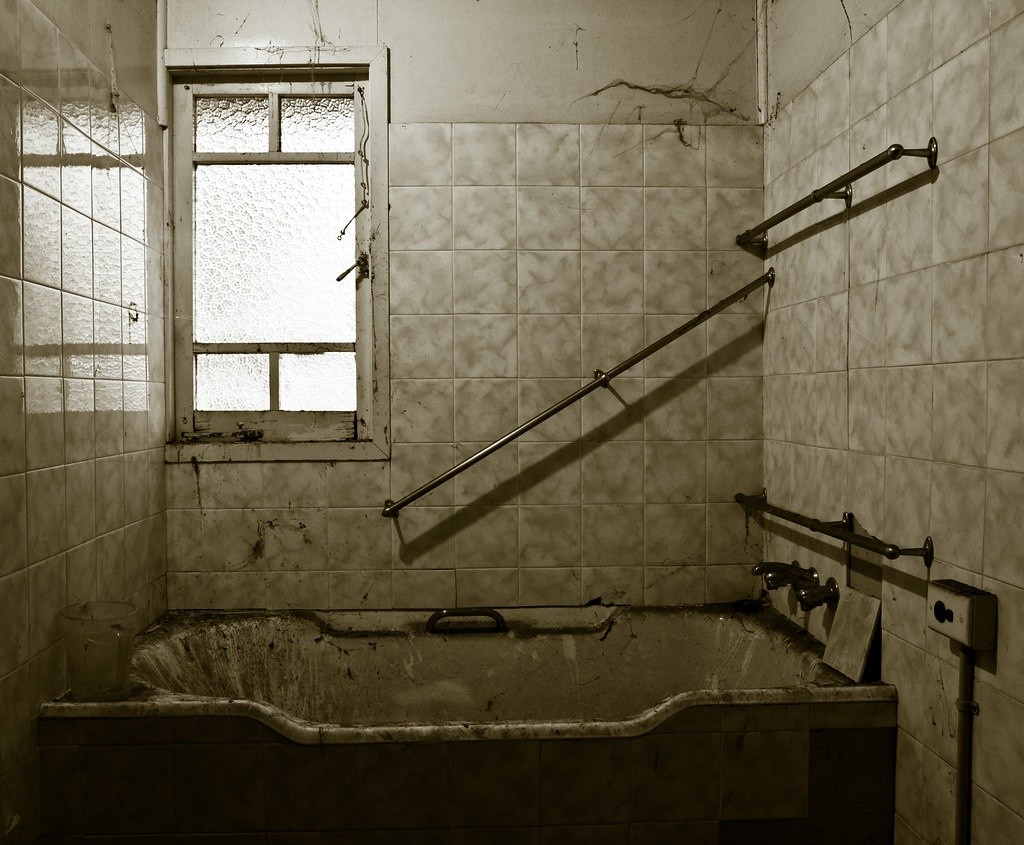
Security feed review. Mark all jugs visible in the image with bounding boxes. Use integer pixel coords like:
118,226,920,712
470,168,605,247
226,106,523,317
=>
60,601,138,702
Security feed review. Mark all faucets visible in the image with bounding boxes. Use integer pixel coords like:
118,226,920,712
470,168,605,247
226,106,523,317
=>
764,567,819,590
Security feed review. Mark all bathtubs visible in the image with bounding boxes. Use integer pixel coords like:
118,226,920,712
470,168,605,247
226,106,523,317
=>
34,595,900,845
